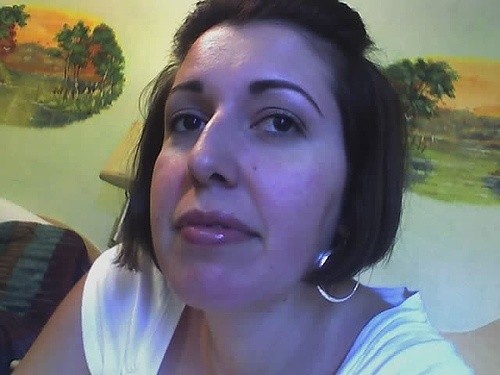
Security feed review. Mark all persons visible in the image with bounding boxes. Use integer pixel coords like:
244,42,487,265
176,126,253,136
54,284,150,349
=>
12,0,480,375
